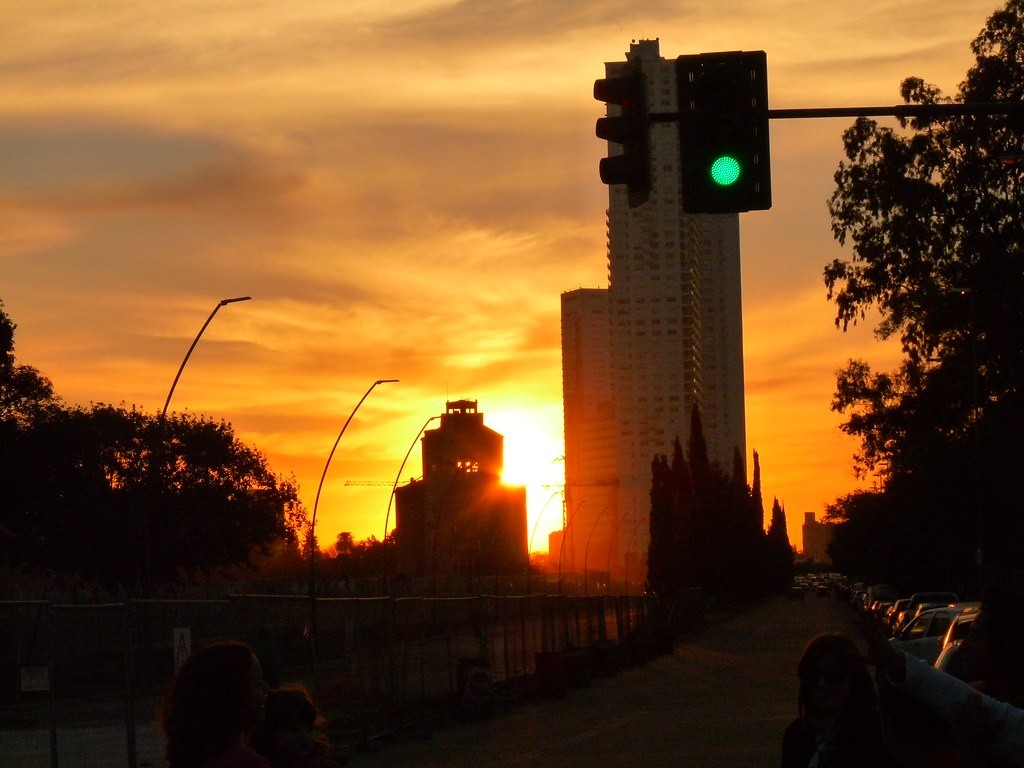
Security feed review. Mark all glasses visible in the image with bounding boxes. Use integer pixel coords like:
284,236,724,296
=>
800,669,857,687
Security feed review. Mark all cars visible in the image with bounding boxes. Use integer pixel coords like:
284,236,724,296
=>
789,566,988,690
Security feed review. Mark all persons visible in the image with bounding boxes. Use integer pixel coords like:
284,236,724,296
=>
775,596,1024,767
161,640,343,767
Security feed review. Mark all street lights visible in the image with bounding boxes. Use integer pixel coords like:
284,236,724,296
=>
148,295,251,435
309,378,404,679
381,412,645,704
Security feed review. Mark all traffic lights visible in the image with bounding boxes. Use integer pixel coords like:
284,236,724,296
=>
593,71,654,208
674,51,772,218
